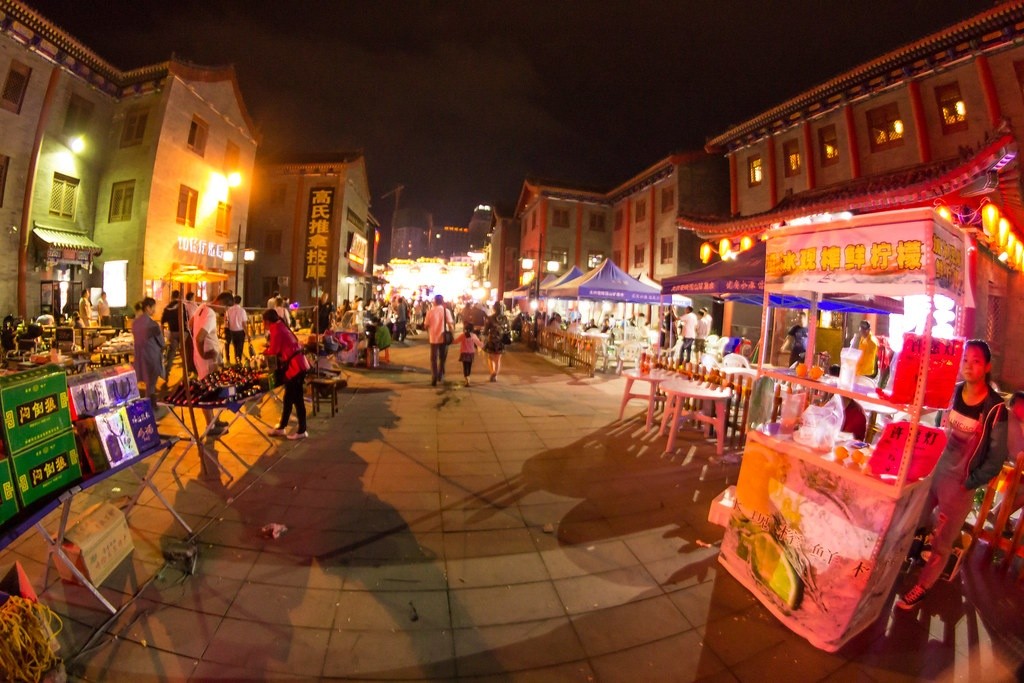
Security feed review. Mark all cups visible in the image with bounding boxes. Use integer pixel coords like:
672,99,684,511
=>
781,392,806,430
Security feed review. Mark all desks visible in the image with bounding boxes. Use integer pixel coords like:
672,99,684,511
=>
0,435,194,615
659,380,734,456
618,367,689,431
155,381,289,480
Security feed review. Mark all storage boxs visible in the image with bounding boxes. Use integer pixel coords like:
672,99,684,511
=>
0,362,161,526
46,500,136,589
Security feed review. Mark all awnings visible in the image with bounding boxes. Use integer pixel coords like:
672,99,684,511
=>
350,263,371,278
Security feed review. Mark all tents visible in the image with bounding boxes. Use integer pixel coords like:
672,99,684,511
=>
502,265,597,322
661,240,902,364
550,258,673,359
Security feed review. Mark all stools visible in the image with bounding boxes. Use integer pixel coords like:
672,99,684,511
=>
310,379,340,418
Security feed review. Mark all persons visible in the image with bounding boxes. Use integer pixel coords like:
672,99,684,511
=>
97,292,111,324
658,304,712,365
896,339,1009,610
27,308,53,340
72,289,92,330
132,302,165,410
856,320,878,379
268,293,291,326
987,394,1024,534
311,292,510,386
160,290,248,435
780,312,815,368
263,309,311,439
511,306,646,342
827,365,867,441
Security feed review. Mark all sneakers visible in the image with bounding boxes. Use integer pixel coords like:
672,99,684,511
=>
897,584,925,609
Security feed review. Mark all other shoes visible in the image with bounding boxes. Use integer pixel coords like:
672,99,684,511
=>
214,418,229,426
287,431,309,439
438,376,442,381
207,426,229,436
490,375,497,383
268,428,286,436
431,381,436,386
465,383,470,387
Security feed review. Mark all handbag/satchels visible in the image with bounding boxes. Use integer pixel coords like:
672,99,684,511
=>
248,341,254,357
502,333,512,345
444,331,453,344
283,308,288,325
